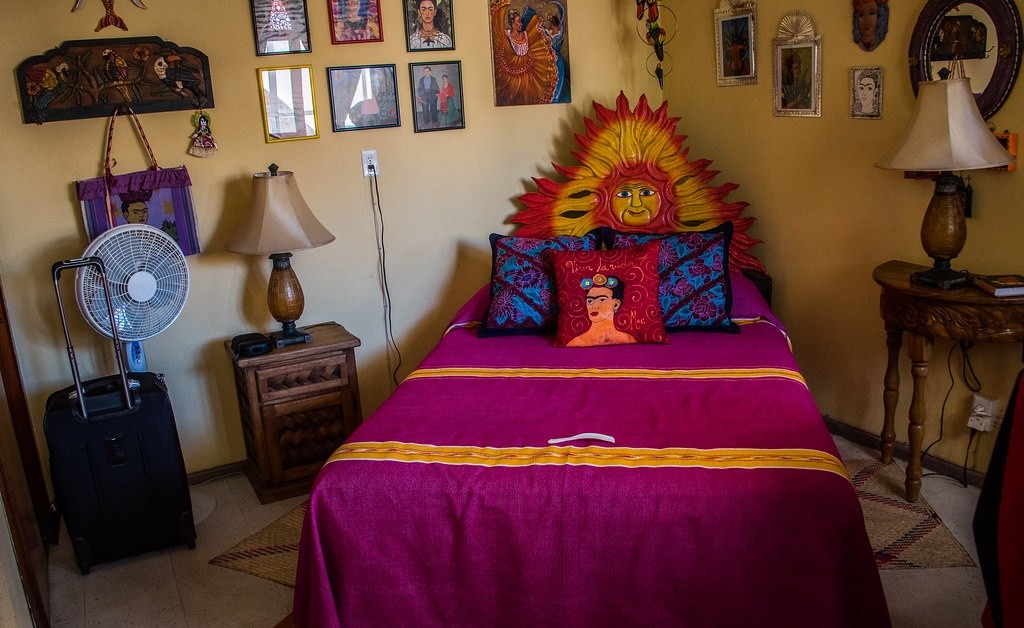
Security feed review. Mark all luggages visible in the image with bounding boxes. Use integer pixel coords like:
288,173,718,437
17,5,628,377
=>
43,256,196,576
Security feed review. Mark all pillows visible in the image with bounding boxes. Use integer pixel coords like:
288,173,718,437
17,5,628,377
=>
599,220,741,334
475,226,607,339
546,239,671,348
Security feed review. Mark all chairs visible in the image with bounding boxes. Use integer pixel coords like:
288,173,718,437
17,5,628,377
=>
971,367,1024,628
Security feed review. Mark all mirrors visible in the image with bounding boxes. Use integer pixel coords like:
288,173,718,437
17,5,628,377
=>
907,0,1024,122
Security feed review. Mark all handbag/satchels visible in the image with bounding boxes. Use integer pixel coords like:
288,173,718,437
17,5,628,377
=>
69,107,204,264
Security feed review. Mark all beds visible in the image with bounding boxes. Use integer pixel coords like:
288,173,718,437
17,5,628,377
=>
273,88,893,628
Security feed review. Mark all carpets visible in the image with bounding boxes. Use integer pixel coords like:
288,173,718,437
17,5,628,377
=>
209,498,307,588
842,455,979,570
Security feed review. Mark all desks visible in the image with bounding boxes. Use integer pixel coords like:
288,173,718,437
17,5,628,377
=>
872,259,1024,502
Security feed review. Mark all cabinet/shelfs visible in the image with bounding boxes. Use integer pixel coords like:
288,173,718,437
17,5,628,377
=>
224,320,362,505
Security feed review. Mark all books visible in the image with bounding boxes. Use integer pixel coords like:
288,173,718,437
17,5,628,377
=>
973,274,1024,297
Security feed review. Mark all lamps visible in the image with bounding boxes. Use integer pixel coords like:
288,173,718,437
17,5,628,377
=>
873,75,1017,291
224,163,336,349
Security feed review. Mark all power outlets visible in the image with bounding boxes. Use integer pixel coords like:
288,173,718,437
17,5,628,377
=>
966,392,1002,433
361,149,380,178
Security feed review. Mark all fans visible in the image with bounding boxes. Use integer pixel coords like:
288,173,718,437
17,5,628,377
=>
73,224,191,372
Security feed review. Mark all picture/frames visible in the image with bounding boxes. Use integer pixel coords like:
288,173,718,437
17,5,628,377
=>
256,63,320,145
848,65,883,120
327,63,401,133
409,59,466,133
771,34,822,118
712,0,760,87
327,0,384,45
249,0,312,56
403,0,457,53
